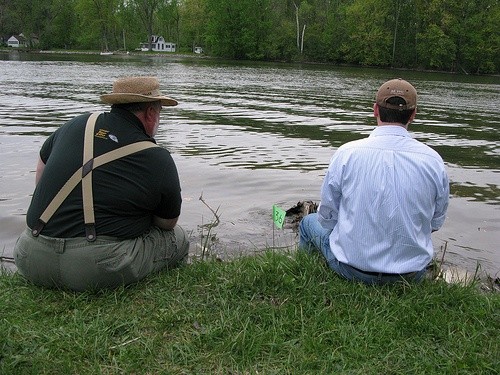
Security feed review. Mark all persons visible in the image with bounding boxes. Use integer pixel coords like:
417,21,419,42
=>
13,76,190,292
299,77,449,287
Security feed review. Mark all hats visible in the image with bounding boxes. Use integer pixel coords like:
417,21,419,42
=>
375,78,418,110
99,76,178,106
